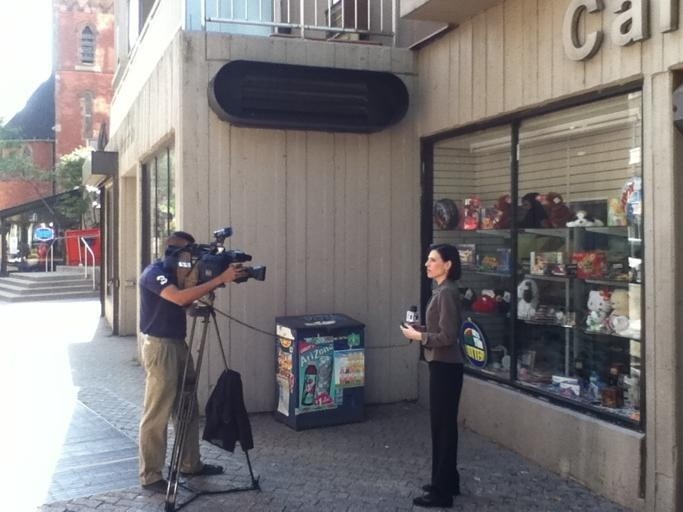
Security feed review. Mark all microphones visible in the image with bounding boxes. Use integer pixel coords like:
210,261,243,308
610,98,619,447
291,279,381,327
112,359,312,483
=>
406,305,417,323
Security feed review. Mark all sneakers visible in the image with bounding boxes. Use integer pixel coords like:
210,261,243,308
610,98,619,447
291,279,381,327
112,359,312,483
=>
422,484,460,495
142,480,173,495
180,463,223,475
414,495,453,507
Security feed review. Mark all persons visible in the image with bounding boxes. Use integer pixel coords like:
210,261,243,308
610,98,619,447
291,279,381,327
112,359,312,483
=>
397,243,464,508
135,230,249,496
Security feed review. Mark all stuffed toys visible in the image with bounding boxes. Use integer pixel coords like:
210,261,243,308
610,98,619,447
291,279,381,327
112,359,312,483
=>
608,289,629,332
564,208,605,228
518,193,553,230
584,290,610,334
462,195,480,230
491,195,512,230
544,191,571,226
587,369,602,401
517,279,540,321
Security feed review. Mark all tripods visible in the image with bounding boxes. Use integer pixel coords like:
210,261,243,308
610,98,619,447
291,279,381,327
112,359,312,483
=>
160,310,262,510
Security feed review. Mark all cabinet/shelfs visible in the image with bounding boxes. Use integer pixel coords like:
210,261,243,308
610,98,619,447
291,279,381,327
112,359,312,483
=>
459,225,641,420
